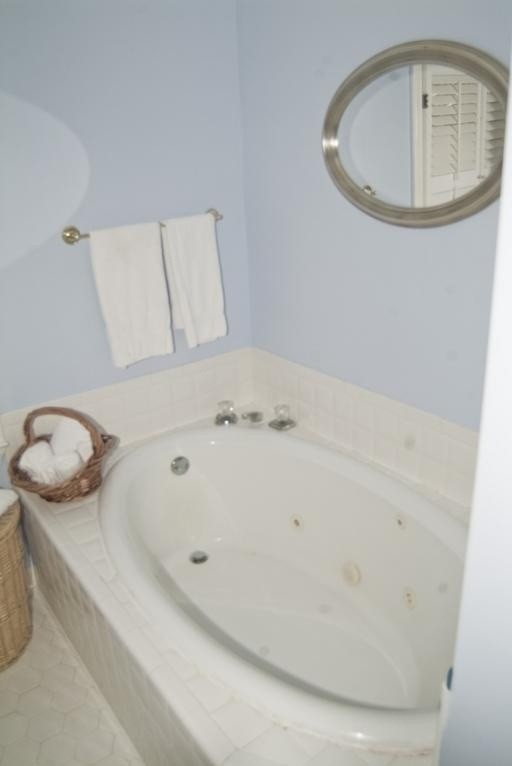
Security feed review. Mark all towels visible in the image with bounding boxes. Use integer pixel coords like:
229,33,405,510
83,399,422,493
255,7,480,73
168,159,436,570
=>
161,213,228,349
89,223,174,369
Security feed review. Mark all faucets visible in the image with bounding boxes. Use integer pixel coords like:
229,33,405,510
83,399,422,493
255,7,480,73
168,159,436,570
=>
241,410,263,422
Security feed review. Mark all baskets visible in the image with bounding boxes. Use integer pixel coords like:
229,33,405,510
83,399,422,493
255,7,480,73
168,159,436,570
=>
7,407,122,508
0,486,36,672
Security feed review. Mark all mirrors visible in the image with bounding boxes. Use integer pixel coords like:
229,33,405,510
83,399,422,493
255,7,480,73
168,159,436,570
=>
320,40,511,229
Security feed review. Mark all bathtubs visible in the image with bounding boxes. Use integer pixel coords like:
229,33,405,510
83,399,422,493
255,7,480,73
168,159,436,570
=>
97,423,470,748
